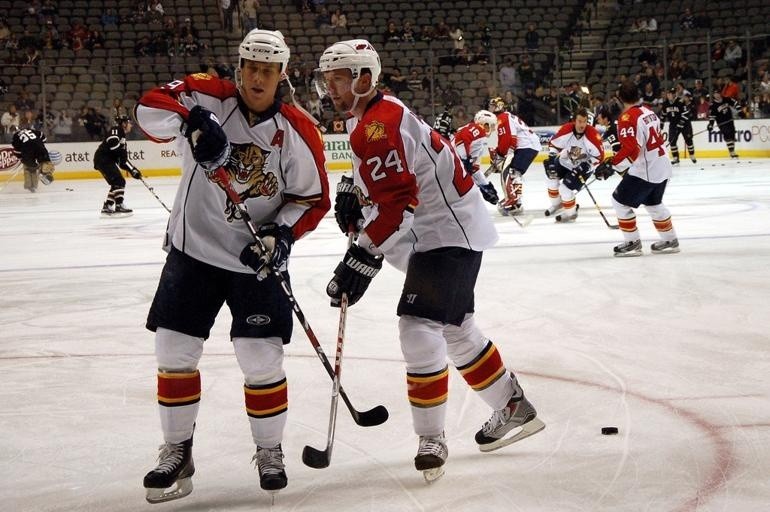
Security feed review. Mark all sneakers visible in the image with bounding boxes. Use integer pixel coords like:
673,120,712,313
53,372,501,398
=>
414,431,448,470
497,198,679,255
690,155,697,163
115,204,132,213
143,438,195,486
671,156,680,164
101,200,115,213
251,444,288,490
730,151,739,157
474,373,537,445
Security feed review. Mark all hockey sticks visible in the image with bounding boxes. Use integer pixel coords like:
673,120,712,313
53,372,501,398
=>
137,173,174,213
574,166,619,230
212,167,388,426
470,172,533,228
303,221,357,467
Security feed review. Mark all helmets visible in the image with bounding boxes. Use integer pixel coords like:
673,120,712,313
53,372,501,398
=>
445,101,453,111
237,29,290,77
314,40,382,99
489,96,505,112
474,109,497,135
114,114,133,127
666,86,677,95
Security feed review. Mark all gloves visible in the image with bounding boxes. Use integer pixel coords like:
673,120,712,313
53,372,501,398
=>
179,105,232,171
478,151,617,204
325,243,384,308
707,120,714,132
335,175,366,240
130,167,143,179
240,221,294,284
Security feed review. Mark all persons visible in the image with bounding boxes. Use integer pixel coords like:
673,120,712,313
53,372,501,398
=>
134,29,332,504
0,1,770,257
313,38,545,483
